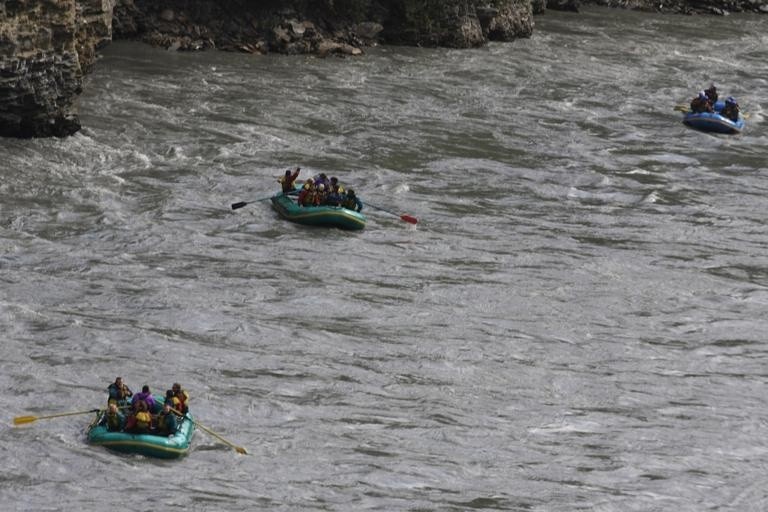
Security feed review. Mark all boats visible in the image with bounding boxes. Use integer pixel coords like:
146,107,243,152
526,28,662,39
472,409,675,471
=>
681,101,745,133
273,185,366,230
87,393,195,460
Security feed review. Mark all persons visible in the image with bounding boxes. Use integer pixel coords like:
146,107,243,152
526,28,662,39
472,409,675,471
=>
277,168,300,193
691,86,740,121
298,173,362,213
106,377,189,438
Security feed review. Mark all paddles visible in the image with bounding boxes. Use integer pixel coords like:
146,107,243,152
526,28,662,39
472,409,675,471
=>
155,400,248,454
13,406,132,425
361,201,417,224
232,189,299,210
